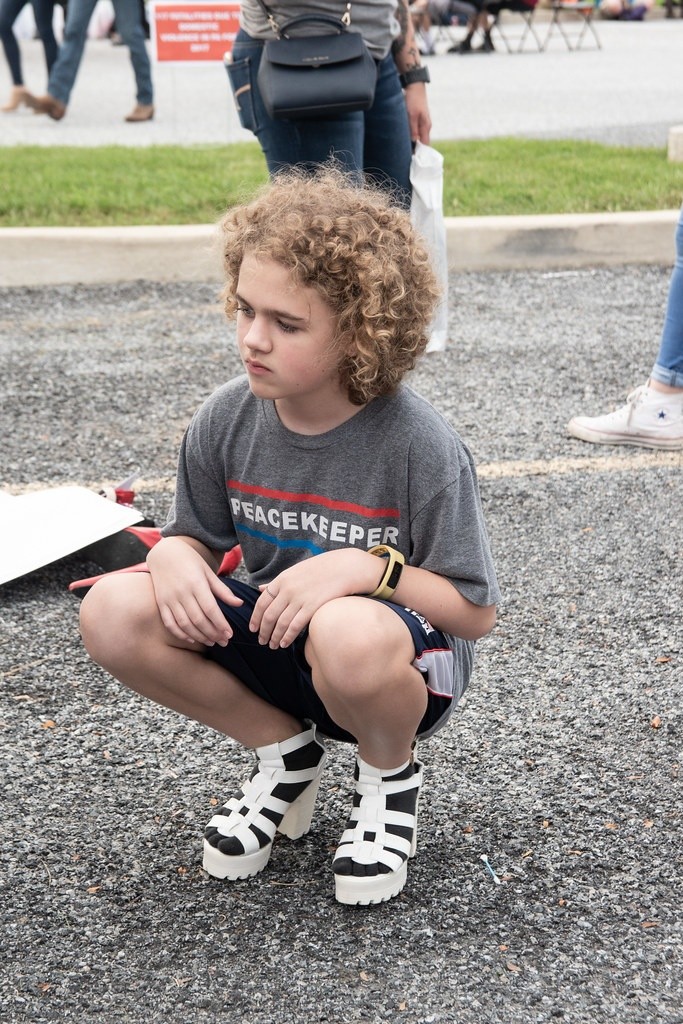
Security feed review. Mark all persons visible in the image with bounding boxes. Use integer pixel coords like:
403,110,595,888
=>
565,203,683,450
81,170,501,907
0,0,154,121
224,0,432,212
409,0,538,54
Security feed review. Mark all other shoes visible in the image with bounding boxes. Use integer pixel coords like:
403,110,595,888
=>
2,89,28,110
448,41,470,52
127,103,153,121
23,93,65,120
476,39,494,50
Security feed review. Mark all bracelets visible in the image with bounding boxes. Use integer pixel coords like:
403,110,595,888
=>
367,545,406,598
399,66,430,87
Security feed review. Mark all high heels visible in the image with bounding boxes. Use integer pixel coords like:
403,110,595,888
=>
334,737,424,905
203,718,327,880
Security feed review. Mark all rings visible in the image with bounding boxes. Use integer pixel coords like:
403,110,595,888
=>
266,586,276,599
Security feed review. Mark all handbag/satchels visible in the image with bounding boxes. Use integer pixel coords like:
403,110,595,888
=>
410,139,449,354
257,14,378,121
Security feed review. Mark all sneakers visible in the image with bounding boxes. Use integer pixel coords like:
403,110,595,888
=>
568,378,683,451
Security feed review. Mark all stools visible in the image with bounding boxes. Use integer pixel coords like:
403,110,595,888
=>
410,0,605,59
